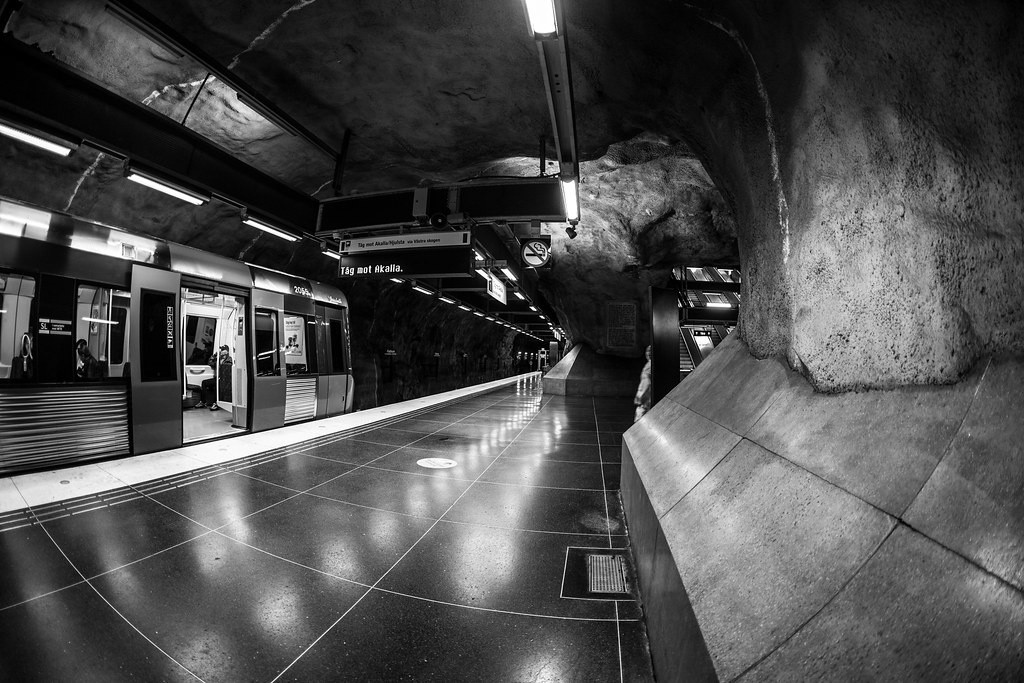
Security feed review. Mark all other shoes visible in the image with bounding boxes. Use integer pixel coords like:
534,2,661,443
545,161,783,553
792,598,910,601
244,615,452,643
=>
210,403,219,411
195,401,207,408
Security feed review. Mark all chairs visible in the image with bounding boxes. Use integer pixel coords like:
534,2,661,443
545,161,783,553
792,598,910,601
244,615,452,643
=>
0,347,316,424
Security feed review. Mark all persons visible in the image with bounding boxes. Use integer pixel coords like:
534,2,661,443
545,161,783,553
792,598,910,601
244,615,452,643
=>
10,332,33,379
194,345,232,411
75,339,102,382
634,345,651,424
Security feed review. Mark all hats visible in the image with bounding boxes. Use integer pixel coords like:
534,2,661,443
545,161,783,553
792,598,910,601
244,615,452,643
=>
219,345,229,352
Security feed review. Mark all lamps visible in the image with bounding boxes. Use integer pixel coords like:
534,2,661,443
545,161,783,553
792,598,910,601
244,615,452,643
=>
565,221,578,239
318,247,568,350
241,213,302,244
0,123,75,156
521,0,564,37
123,165,211,207
558,175,580,221
